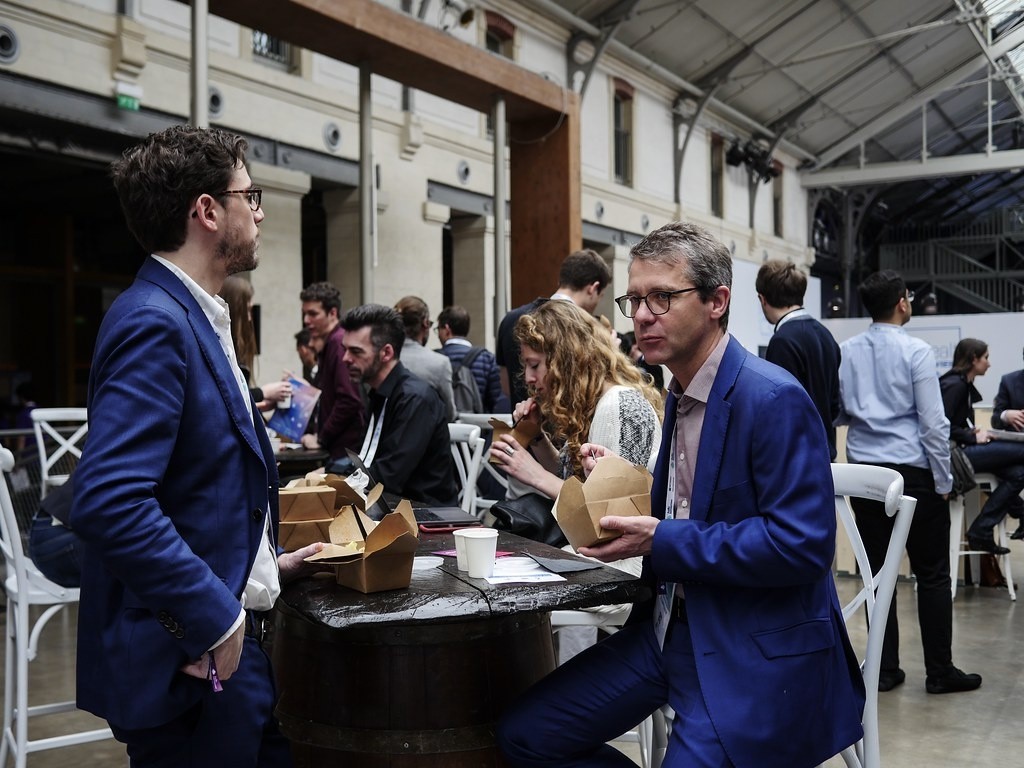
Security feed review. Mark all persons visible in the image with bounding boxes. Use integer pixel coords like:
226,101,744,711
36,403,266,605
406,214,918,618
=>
593,315,664,395
28,476,86,588
497,222,867,768
339,304,458,508
218,276,369,487
491,300,666,668
0,383,50,471
833,270,982,695
497,247,612,428
393,295,501,422
939,339,1024,590
755,259,842,462
76,121,324,768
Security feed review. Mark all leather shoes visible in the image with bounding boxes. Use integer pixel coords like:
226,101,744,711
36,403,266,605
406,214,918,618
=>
969,534,1010,554
925,667,981,693
878,669,905,691
1012,516,1024,541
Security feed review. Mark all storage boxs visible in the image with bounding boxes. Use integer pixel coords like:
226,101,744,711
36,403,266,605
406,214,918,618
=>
278,471,422,595
551,457,653,554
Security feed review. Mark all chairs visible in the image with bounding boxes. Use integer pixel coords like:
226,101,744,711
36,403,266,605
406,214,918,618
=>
447,403,1024,768
0,443,116,768
28,406,89,617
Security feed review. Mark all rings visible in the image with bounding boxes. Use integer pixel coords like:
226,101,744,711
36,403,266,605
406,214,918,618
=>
505,447,514,456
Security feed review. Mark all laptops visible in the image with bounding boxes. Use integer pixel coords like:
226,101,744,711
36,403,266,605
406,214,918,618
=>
345,447,481,526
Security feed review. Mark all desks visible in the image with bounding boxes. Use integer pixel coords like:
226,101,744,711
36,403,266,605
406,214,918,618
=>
275,492,652,768
276,448,331,469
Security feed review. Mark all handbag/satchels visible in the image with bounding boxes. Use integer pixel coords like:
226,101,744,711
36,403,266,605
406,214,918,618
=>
947,439,977,496
965,554,1018,591
493,493,565,549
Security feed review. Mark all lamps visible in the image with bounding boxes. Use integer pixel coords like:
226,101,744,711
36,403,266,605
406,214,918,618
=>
725,137,783,184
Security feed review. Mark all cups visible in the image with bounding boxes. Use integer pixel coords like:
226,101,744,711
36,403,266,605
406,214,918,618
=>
277,388,291,408
453,528,499,578
269,438,281,455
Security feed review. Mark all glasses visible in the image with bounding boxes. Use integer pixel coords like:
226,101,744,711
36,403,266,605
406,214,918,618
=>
892,290,915,310
615,283,721,318
192,186,262,219
433,327,442,334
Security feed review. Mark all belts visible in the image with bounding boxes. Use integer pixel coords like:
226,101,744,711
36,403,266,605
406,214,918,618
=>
246,609,269,642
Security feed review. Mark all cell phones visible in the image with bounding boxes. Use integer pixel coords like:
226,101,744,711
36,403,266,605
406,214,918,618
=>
420,523,484,532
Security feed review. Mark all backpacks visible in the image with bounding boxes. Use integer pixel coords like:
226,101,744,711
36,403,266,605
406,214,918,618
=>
434,348,485,414
